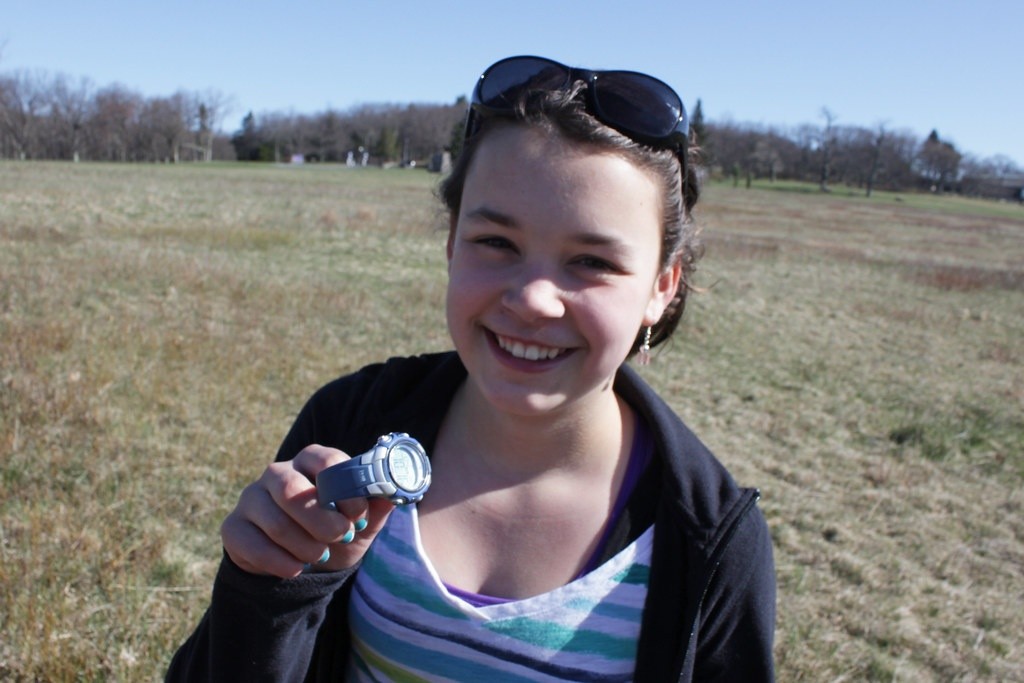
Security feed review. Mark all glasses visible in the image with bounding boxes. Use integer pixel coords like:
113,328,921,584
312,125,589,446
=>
461,55,689,195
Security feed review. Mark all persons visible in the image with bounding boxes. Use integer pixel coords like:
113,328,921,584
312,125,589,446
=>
161,53,777,683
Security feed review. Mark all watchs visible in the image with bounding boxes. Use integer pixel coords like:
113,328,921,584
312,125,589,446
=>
315,432,433,507
